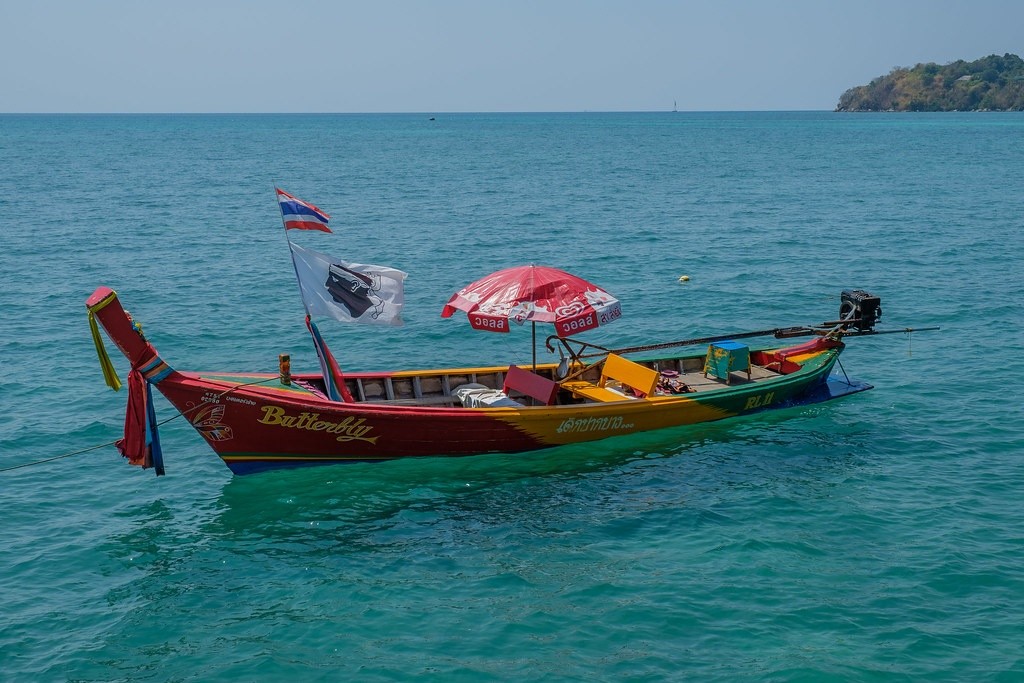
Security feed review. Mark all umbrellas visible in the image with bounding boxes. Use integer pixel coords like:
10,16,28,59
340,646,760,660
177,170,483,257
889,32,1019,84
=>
441,262,622,374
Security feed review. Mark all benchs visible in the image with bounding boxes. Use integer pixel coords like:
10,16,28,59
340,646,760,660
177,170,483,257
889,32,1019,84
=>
555,353,660,402
461,364,559,407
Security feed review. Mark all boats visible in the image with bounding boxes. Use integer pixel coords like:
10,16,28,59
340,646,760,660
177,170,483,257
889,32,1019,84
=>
84,193,942,478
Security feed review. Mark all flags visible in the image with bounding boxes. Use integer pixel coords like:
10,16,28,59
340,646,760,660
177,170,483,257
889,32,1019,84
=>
276,188,333,234
289,242,408,326
